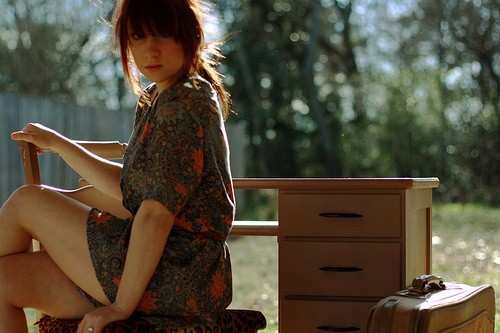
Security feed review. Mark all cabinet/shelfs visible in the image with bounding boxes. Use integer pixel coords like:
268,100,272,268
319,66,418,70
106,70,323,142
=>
233,176,439,333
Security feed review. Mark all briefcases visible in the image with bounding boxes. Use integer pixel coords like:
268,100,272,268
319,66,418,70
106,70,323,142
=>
364,274,496,332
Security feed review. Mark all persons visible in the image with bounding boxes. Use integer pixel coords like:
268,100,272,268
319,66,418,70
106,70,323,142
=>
0,0,235,333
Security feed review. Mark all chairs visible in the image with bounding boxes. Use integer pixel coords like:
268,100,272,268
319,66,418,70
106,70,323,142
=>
18,139,267,333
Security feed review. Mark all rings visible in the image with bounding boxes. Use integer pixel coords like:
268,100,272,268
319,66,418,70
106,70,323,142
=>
88,328,94,331
